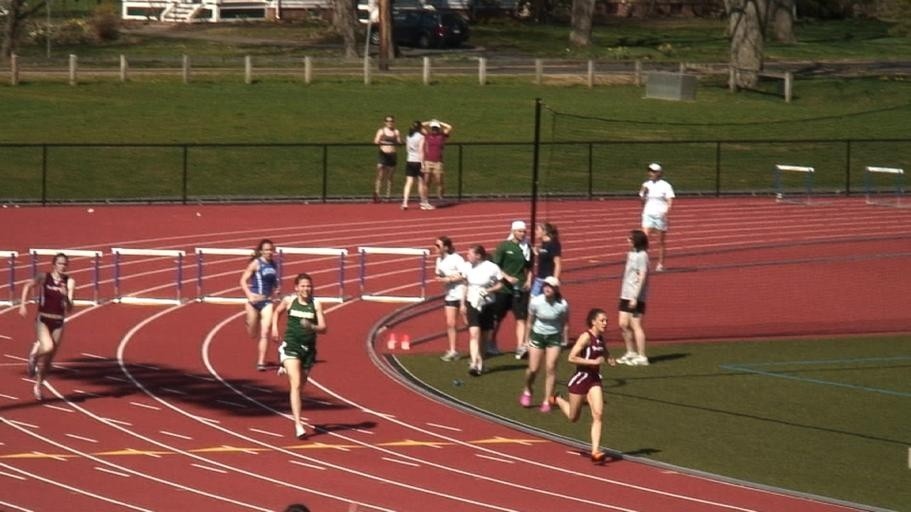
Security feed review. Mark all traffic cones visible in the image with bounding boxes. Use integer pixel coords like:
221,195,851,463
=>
401,335,413,353
383,330,399,352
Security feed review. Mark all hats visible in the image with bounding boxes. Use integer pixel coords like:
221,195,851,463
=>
538,276,561,288
429,122,441,128
648,163,662,172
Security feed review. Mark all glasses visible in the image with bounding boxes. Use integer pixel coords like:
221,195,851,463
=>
434,242,443,249
385,120,394,123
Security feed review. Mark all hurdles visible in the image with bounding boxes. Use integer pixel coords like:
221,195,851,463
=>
356,245,432,304
276,247,349,303
29,248,103,307
1,250,22,308
109,247,187,306
192,247,271,303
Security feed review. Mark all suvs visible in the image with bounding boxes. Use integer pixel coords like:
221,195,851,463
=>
388,3,469,47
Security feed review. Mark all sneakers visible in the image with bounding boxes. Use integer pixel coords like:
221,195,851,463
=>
615,352,636,364
591,453,612,464
442,335,562,417
296,422,306,437
419,203,435,210
625,355,650,366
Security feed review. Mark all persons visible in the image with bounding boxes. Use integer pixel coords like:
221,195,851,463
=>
20,254,75,402
535,226,565,274
372,115,402,197
519,275,571,411
548,307,615,462
462,246,504,374
241,238,280,369
423,120,451,207
487,221,530,361
640,161,678,274
272,273,328,441
610,229,655,368
401,122,436,211
432,235,465,362
